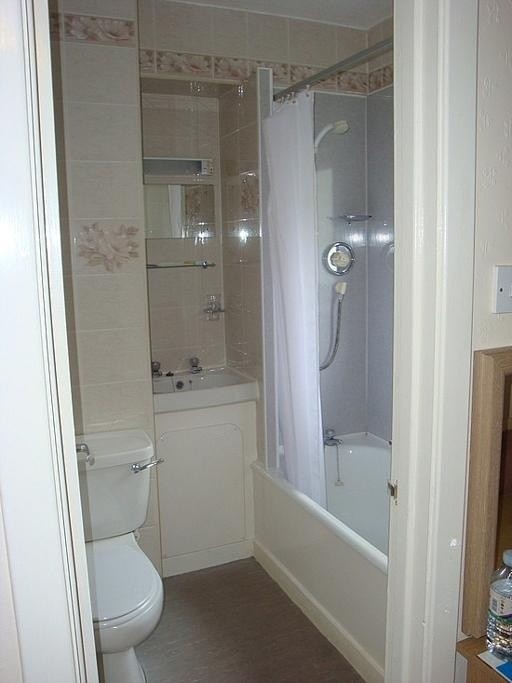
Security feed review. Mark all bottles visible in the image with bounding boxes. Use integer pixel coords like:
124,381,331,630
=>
486,549,512,662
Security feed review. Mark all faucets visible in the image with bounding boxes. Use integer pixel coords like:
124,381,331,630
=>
324,428,344,447
152,362,162,377
188,356,202,374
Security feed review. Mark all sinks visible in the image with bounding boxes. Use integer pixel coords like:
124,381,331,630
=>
152,374,249,393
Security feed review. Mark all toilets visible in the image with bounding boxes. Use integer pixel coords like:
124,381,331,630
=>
74,428,164,682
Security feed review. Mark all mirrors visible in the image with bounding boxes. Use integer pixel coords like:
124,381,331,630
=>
143,184,215,239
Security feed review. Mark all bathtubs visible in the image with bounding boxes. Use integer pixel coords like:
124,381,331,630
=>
252,430,391,682
